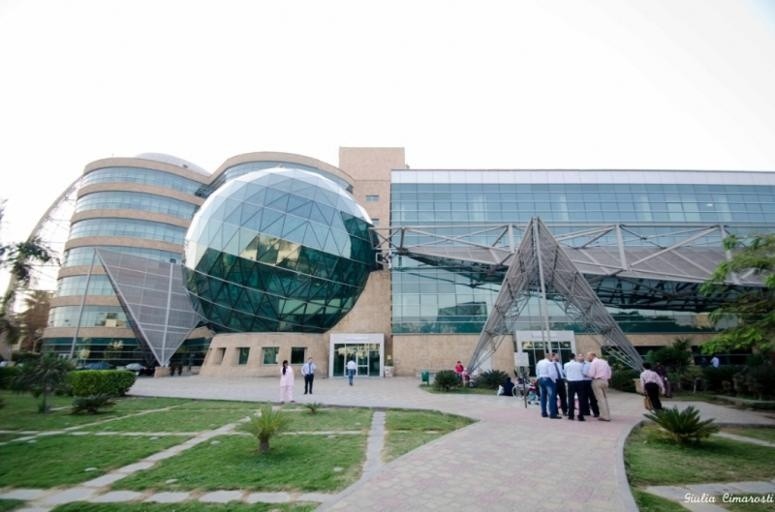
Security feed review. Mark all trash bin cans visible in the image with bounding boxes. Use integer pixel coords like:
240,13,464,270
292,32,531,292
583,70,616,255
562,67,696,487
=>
422,372,430,387
633,379,641,393
384,366,395,377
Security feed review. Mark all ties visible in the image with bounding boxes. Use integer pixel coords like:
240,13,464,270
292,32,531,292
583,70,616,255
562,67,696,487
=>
309,364,311,374
554,363,561,379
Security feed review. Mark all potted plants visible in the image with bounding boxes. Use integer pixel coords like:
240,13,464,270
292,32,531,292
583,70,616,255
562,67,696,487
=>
383,354,395,379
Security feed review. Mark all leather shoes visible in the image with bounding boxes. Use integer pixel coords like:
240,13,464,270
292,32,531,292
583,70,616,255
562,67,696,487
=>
542,413,610,421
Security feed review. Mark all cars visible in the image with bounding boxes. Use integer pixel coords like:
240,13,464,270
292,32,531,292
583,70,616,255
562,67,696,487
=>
76,362,146,377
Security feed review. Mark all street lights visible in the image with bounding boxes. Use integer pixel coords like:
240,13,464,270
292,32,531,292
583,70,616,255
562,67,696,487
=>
160,258,175,367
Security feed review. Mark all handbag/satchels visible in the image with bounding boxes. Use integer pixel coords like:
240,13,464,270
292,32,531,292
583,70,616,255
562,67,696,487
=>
645,396,653,409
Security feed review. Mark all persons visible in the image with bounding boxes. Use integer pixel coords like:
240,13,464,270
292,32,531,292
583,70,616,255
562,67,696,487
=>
536,351,613,420
454,359,465,373
279,359,295,403
710,353,720,368
526,377,540,405
640,361,665,416
301,356,316,394
347,356,356,386
654,360,673,397
503,376,514,395
